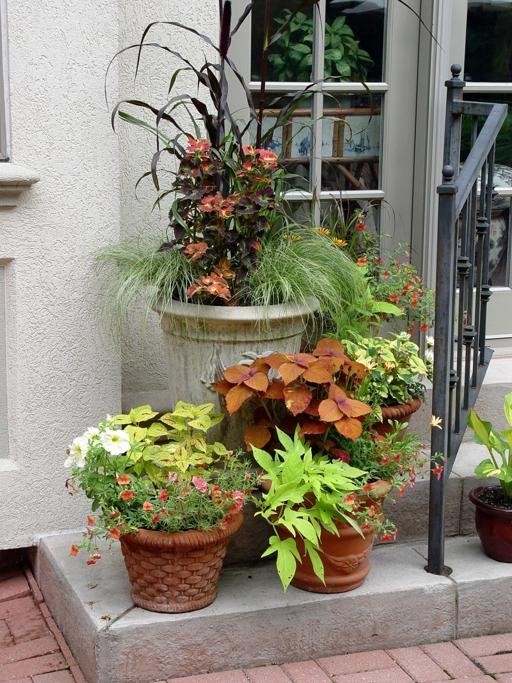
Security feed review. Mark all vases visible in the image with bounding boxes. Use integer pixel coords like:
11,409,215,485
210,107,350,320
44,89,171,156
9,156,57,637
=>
119,511,244,614
146,290,318,468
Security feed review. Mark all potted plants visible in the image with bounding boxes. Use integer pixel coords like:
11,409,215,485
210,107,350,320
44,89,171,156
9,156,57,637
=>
212,330,429,460
467,390,512,563
246,421,386,593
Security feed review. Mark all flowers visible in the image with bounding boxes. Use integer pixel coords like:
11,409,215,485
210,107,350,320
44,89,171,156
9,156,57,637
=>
264,414,445,544
105,0,372,300
63,400,261,566
349,202,438,332
